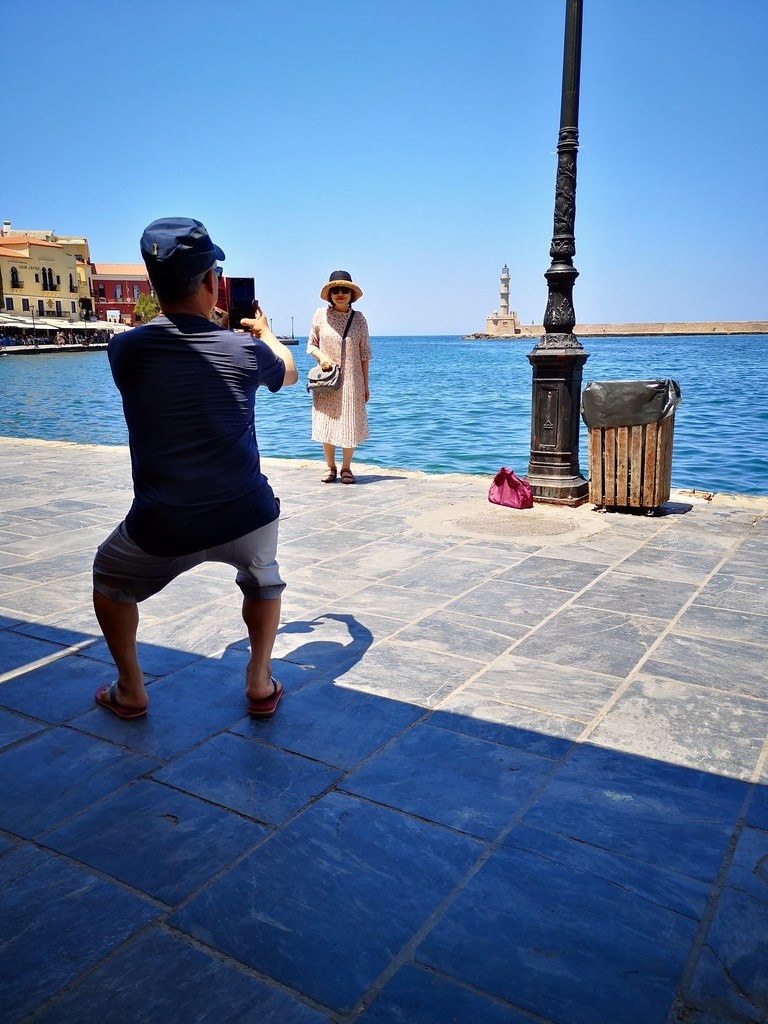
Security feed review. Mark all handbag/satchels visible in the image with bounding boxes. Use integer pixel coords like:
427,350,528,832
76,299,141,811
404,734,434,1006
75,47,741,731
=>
488,467,533,508
306,364,340,391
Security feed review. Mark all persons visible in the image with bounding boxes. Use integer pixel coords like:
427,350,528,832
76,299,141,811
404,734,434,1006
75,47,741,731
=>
91,217,298,719
0,329,114,349
306,271,372,484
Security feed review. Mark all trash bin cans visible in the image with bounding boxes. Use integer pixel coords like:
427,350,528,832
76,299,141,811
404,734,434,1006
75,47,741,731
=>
3,338,16,346
585,378,681,516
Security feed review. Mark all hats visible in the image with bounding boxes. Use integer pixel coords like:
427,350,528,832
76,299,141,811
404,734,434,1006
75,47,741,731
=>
140,216,226,279
321,271,363,302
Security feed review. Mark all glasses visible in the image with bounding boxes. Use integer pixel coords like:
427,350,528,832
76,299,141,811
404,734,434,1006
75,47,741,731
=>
203,266,223,283
330,287,350,294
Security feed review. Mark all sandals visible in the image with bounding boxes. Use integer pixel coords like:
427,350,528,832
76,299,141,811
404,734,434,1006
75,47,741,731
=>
340,469,355,484
321,467,337,482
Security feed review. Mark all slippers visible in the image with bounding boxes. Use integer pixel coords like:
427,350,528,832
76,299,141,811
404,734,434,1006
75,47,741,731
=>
95,680,148,720
247,676,282,716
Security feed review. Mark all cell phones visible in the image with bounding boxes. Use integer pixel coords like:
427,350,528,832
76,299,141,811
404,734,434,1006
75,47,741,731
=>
227,277,256,332
